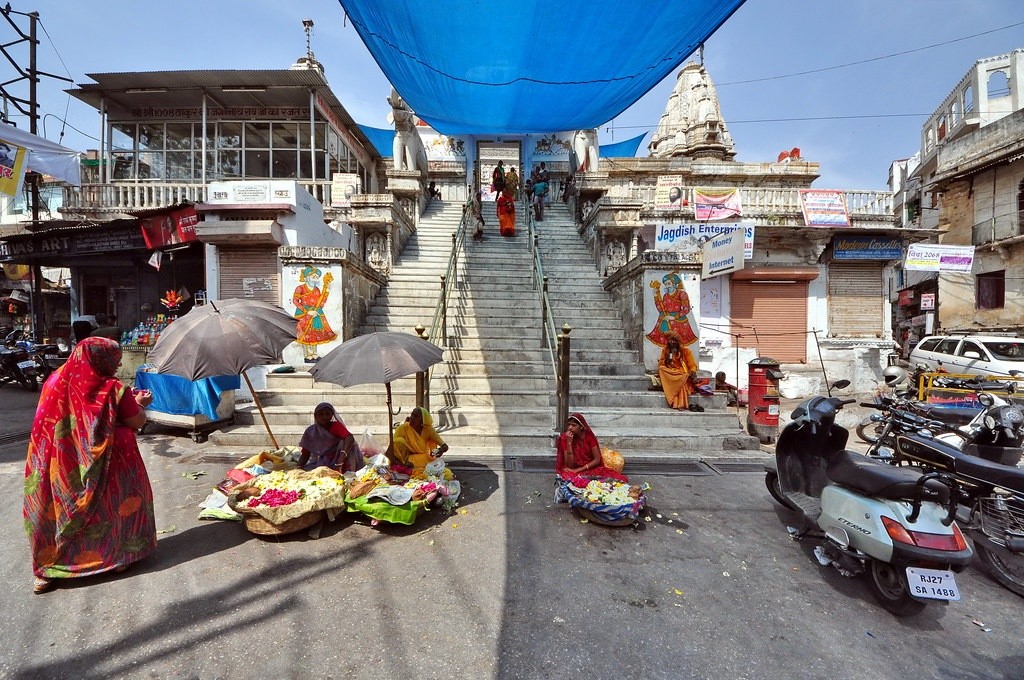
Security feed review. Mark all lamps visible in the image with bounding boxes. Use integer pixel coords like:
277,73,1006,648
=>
222,87,266,91
126,88,169,93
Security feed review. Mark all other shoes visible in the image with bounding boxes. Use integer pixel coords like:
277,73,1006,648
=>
546,204,550,207
33,576,57,594
689,403,697,412
695,404,704,412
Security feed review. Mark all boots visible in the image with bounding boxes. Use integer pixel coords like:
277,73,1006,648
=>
534,204,540,221
540,205,544,221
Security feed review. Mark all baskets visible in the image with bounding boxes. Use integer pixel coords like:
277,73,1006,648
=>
228,473,346,535
563,475,640,525
354,464,438,517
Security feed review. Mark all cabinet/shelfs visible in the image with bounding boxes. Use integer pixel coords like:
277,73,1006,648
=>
194,291,208,307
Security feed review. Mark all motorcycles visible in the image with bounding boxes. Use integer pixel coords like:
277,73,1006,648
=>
0,339,42,391
5,328,60,381
856,365,1024,597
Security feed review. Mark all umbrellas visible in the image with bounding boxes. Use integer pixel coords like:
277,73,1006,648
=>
308,322,445,461
150,299,301,451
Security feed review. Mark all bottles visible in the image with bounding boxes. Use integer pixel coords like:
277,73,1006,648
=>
120,315,178,346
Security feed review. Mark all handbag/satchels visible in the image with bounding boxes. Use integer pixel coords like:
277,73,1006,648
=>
491,183,496,192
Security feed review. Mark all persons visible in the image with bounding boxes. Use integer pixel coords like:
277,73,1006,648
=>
556,413,627,482
473,214,484,242
297,403,364,474
530,174,549,221
658,331,697,408
496,188,515,235
21,337,159,594
429,182,441,200
526,162,550,207
715,372,745,408
669,187,681,206
0,144,14,169
493,160,506,201
506,167,518,201
392,406,448,472
72,312,122,345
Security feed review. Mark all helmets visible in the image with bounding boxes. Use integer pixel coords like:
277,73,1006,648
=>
882,365,907,387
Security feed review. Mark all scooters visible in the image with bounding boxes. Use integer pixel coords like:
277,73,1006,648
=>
763,369,974,622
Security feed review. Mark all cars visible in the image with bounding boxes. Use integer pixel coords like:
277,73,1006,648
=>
909,334,1024,390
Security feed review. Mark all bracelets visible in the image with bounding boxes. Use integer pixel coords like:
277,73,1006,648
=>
334,462,344,466
340,450,347,457
137,403,145,409
586,464,589,469
567,452,573,454
